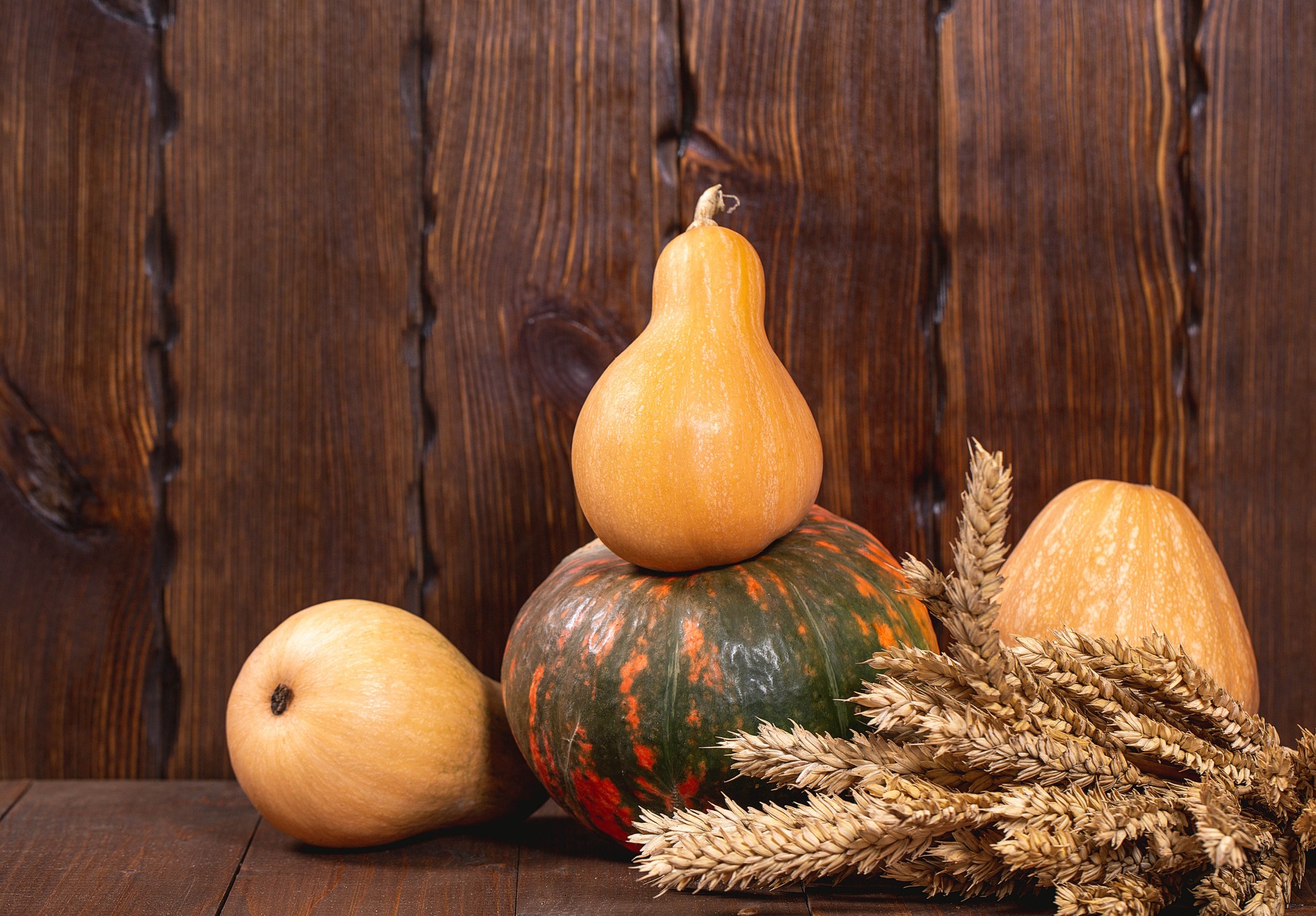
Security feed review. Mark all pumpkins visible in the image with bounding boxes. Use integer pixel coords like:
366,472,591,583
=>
225,184,1260,850
